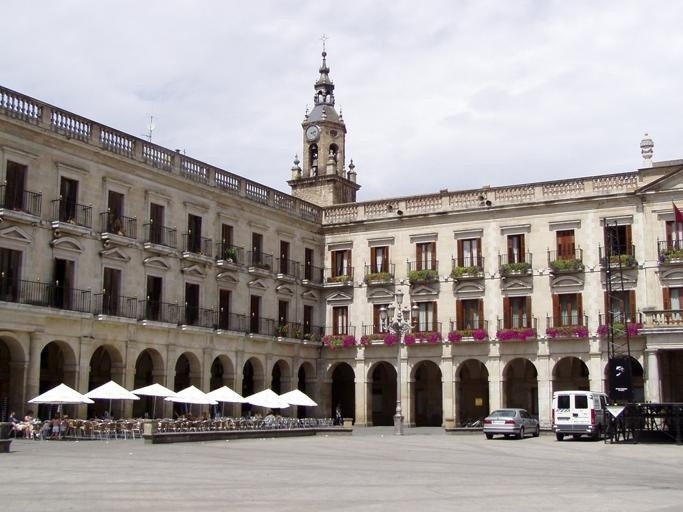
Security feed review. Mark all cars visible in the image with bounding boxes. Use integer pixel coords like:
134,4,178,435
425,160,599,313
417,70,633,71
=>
482,407,540,438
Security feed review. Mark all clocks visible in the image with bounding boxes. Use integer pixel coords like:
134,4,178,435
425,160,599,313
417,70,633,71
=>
306,125,319,142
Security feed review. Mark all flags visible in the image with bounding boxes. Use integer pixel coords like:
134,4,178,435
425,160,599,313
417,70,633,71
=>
673,201,682,226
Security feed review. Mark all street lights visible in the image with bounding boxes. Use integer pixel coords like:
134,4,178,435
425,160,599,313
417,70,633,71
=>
378,289,422,435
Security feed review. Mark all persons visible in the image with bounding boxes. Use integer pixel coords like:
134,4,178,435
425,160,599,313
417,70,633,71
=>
175,409,283,429
8,410,69,443
335,405,343,426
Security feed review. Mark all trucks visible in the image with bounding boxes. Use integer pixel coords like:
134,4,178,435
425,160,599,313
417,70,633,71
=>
550,389,613,440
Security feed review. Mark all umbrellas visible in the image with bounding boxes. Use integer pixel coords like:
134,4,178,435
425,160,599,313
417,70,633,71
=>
204,384,247,416
27,382,95,420
82,380,141,416
130,382,176,418
244,388,291,415
163,385,219,417
280,388,318,418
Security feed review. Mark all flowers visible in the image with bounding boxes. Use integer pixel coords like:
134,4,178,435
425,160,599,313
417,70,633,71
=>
359,333,398,348
320,334,355,352
597,322,644,336
545,327,588,339
448,329,485,343
404,332,440,345
496,328,534,340
